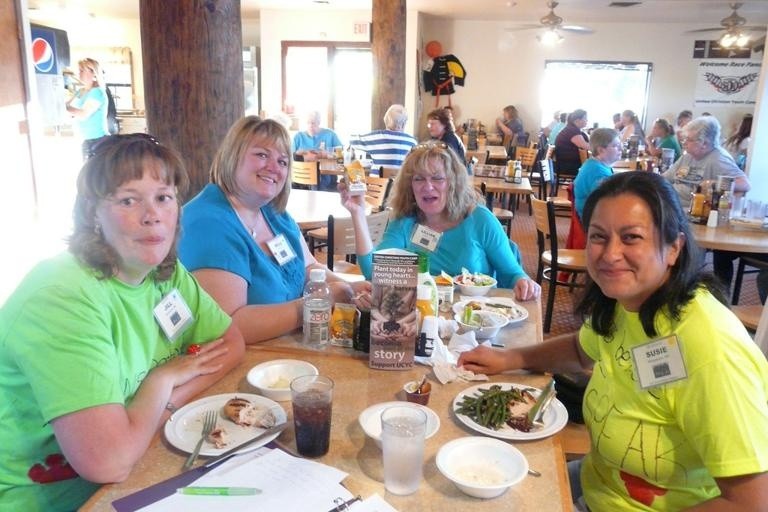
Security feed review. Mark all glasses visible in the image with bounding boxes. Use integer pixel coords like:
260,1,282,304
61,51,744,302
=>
411,143,454,153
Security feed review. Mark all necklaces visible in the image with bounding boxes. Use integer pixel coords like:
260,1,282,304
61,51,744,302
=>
234,203,262,239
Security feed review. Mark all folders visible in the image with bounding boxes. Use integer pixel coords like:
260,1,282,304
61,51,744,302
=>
111,440,362,511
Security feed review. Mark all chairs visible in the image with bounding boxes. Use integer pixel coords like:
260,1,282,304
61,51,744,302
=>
569,271,578,292
530,194,593,333
522,141,538,172
731,304,764,334
481,182,513,239
560,422,591,462
537,158,572,243
466,150,490,164
545,143,576,198
291,161,321,191
364,176,393,214
578,148,588,165
515,146,539,184
379,166,399,198
732,257,768,306
307,210,390,272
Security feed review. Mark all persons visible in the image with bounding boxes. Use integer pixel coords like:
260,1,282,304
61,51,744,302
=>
426,109,468,170
572,128,623,226
178,115,373,344
553,110,590,174
457,171,768,512
675,113,690,149
0,132,246,512
543,111,560,146
343,105,418,199
292,113,343,190
66,58,111,162
721,114,753,162
621,110,645,144
337,139,543,301
547,113,568,159
64,69,120,134
614,114,624,140
647,119,681,163
496,106,525,156
661,118,750,208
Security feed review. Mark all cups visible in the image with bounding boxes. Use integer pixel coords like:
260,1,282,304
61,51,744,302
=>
288,373,335,459
637,144,646,157
380,404,428,497
403,379,432,406
729,194,746,220
747,197,761,220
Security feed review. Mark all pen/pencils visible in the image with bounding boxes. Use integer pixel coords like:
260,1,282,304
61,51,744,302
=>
175,487,264,497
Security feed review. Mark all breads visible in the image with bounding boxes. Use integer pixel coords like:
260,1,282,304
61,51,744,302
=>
226,399,256,425
209,427,231,446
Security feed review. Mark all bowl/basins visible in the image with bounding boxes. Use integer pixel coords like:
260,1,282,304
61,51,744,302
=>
245,357,320,403
454,308,509,340
358,399,442,452
434,436,530,501
452,273,498,298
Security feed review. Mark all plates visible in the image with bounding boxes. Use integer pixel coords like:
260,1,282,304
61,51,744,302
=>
163,393,288,459
451,381,571,442
452,297,530,327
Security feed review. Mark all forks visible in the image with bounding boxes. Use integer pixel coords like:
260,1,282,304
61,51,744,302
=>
181,410,219,475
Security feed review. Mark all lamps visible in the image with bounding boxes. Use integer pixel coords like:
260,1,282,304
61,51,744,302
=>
540,1,563,47
716,2,746,48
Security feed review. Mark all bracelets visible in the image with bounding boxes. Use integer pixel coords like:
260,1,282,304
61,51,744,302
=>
164,401,178,415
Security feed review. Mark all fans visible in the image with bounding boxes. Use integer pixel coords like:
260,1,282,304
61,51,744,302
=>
687,17,767,46
506,23,593,42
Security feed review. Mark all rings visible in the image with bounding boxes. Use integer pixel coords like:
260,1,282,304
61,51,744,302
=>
186,343,202,354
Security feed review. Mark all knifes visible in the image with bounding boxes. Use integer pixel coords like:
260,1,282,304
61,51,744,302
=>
203,421,291,471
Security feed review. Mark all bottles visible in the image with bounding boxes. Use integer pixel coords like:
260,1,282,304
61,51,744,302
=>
505,160,515,182
728,191,734,224
414,251,439,335
691,185,705,215
335,146,343,171
719,191,730,227
514,162,522,183
302,268,331,351
414,286,435,356
